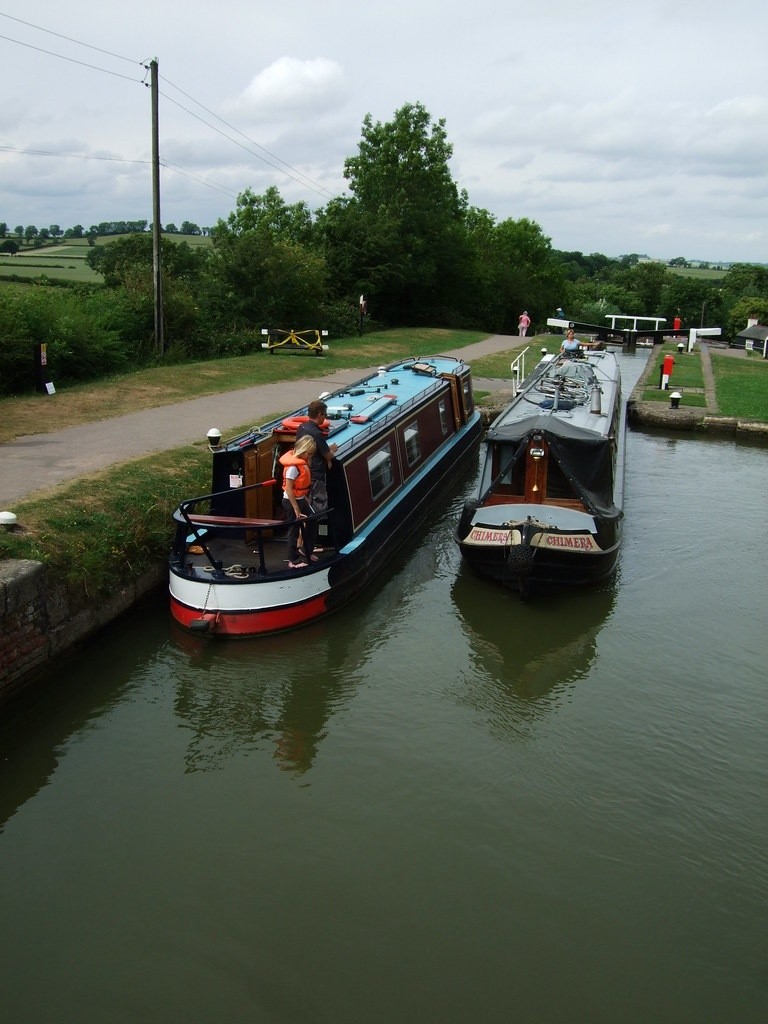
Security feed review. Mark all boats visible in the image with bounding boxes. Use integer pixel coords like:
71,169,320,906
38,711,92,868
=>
452,337,629,605
163,353,484,647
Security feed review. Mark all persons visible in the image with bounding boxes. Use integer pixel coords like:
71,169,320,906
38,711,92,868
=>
553,308,565,334
296,400,338,552
560,329,596,353
518,311,530,337
672,315,684,338
281,435,318,568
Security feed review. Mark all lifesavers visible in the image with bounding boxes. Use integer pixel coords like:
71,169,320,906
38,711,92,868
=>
282,415,331,430
